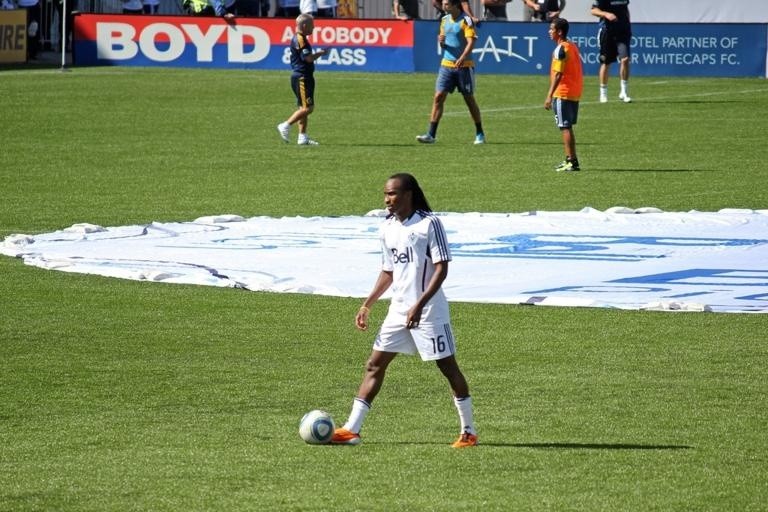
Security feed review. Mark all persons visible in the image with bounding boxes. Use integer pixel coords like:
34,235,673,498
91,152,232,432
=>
480,0,513,22
413,0,489,145
541,16,591,174
276,11,322,146
118,1,489,23
591,0,638,103
326,172,483,450
521,0,567,25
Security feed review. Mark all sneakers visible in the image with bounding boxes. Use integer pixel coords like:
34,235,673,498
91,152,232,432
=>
417,135,436,143
297,138,319,145
452,431,477,447
474,134,486,145
600,93,608,103
619,92,632,103
555,158,578,172
333,427,360,445
277,124,288,141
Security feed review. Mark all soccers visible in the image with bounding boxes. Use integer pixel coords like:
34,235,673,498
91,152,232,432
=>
299,410,335,445
